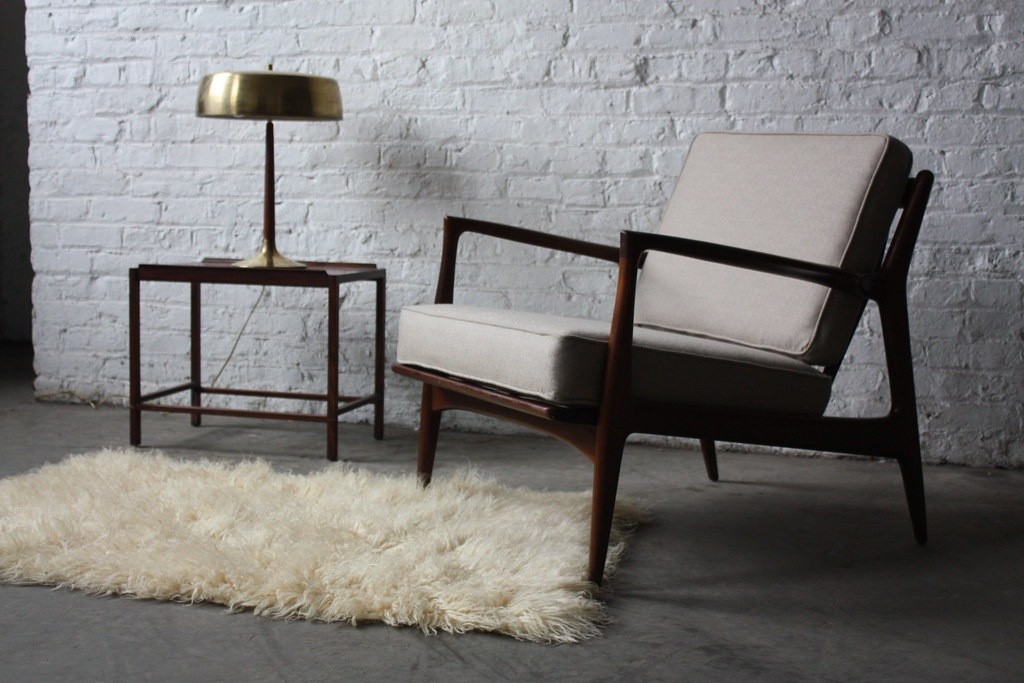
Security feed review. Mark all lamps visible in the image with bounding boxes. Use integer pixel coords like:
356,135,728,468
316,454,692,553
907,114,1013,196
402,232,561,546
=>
195,65,344,272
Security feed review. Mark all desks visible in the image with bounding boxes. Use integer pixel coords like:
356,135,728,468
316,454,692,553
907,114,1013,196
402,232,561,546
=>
129,257,386,462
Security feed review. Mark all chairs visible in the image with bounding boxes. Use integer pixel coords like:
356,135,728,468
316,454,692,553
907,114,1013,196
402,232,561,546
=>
392,131,936,587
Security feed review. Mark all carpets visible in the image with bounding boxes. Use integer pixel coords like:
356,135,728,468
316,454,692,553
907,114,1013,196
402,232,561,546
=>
1,446,654,644
